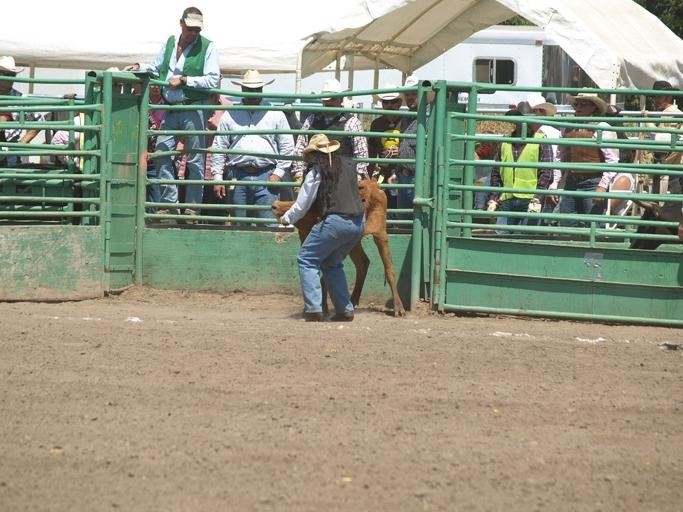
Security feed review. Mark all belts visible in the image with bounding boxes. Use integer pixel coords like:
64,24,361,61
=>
241,167,261,173
172,100,191,105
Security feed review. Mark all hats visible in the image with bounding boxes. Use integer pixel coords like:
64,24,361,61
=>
185,13,204,31
232,69,276,89
400,76,422,93
511,95,557,115
373,86,404,101
567,92,607,115
303,134,341,154
653,80,679,91
313,79,351,101
505,101,541,115
0,56,24,73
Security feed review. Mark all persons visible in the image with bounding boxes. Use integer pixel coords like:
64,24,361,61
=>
1,7,682,245
280,133,364,321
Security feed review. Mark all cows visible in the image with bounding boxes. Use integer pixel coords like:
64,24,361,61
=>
271,178,406,322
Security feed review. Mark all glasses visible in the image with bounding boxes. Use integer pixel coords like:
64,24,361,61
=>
573,100,591,106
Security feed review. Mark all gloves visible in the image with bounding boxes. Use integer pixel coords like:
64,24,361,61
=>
485,200,498,211
275,223,294,242
527,198,542,212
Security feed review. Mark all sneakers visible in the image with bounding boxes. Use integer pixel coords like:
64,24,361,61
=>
331,311,355,320
148,209,179,223
176,211,199,224
292,310,323,321
